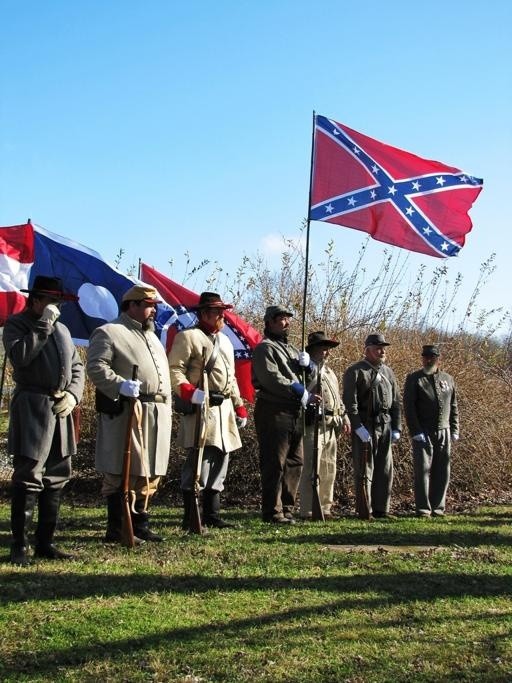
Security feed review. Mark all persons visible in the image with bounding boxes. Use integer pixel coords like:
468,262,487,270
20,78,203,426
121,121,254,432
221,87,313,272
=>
300,331,352,523
341,334,404,520
251,305,318,524
2,275,85,569
402,345,460,519
167,291,248,533
85,284,172,545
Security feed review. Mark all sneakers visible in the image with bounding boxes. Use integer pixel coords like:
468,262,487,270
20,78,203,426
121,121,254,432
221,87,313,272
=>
275,512,446,525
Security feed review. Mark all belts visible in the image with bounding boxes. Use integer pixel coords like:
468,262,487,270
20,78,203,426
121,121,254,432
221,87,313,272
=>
138,394,166,404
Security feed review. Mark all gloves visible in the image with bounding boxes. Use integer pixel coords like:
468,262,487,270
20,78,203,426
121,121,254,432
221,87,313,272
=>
413,433,426,443
392,430,400,444
299,352,310,367
52,390,77,418
191,388,205,404
354,426,371,442
237,417,247,428
451,434,459,442
301,388,309,410
119,378,142,397
39,302,61,326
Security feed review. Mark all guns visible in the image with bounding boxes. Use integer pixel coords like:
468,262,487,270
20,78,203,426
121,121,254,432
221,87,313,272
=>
311,362,324,519
120,365,138,549
188,347,207,534
358,388,370,519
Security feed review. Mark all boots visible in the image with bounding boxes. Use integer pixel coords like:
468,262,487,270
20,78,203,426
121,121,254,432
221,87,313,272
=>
10,489,36,565
35,487,75,560
105,490,237,546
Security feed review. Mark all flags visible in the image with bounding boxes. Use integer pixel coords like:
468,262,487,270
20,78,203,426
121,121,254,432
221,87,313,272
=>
16,223,178,351
309,115,483,259
0,224,34,328
138,264,264,402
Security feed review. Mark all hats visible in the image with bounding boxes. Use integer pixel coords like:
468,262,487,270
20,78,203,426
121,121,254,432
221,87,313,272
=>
422,345,440,357
123,285,162,304
191,292,233,311
20,275,79,304
306,331,340,350
264,306,293,321
365,334,390,346
